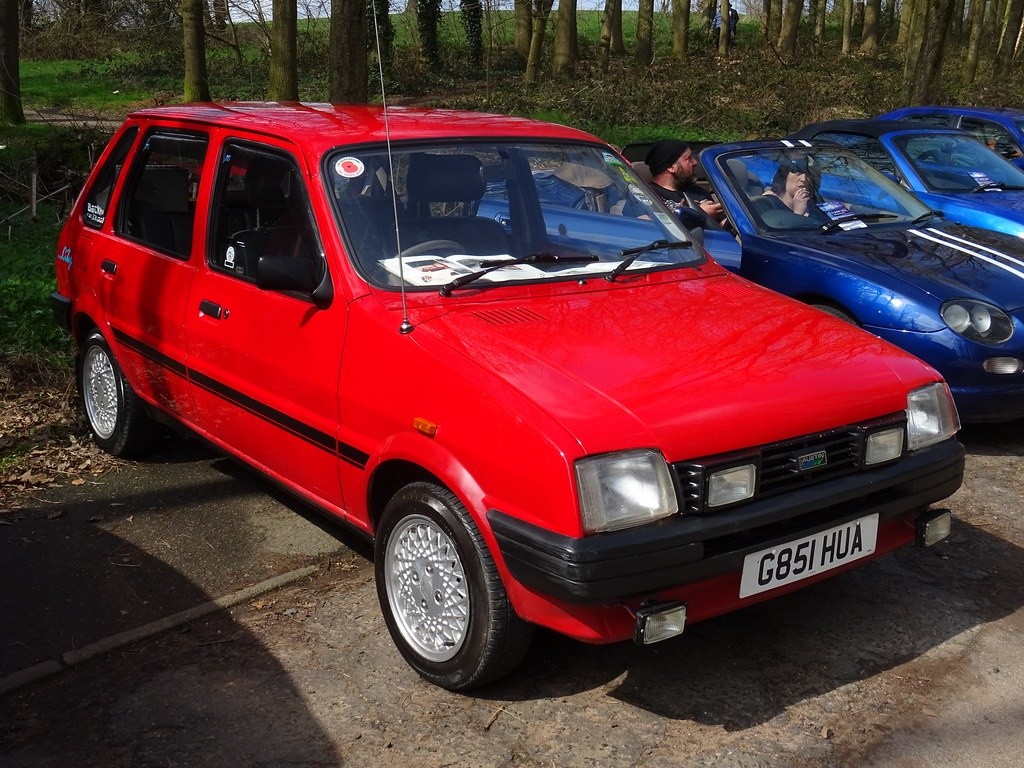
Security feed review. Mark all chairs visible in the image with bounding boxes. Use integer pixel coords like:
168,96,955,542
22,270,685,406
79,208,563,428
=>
632,162,764,203
130,153,510,293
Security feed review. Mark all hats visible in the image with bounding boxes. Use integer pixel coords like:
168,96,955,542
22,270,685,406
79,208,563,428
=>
644,140,689,177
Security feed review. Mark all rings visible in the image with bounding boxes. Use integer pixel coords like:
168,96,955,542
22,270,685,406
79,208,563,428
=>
803,194,806,197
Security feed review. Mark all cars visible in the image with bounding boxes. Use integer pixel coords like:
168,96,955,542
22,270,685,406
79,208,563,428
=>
55,101,977,690
880,105,1024,168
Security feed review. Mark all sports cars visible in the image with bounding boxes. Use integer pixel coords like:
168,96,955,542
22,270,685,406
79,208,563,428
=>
480,136,1024,410
788,117,1024,239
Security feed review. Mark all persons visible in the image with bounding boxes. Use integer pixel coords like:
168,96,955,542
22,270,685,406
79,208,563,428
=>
622,138,727,230
762,154,822,217
711,3,739,52
